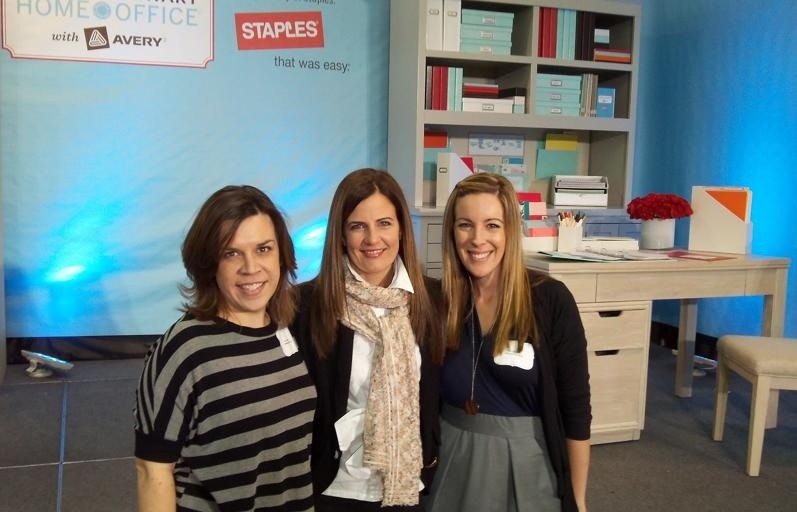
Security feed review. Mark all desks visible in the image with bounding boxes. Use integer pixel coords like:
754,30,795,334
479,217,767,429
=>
525,250,789,446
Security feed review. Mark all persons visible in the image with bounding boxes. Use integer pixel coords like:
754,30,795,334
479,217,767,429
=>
431,173,592,512
286,167,446,510
132,185,317,511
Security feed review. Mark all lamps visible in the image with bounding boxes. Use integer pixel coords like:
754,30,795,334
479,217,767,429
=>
21,350,74,378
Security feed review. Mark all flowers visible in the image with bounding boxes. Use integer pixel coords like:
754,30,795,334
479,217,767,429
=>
628,192,693,219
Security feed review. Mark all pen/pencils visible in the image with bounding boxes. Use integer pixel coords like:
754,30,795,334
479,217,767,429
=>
557,209,586,227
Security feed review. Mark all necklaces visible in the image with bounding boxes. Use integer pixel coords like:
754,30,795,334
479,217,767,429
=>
465,297,498,416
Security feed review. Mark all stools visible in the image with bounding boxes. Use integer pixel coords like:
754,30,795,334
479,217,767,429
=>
713,334,795,478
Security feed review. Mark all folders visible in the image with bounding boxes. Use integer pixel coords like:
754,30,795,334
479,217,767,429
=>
535,246,674,262
435,152,475,209
687,185,753,255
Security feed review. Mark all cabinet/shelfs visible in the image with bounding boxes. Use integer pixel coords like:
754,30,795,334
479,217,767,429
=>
389,0,638,213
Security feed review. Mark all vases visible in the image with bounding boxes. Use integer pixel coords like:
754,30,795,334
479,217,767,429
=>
628,218,694,249
